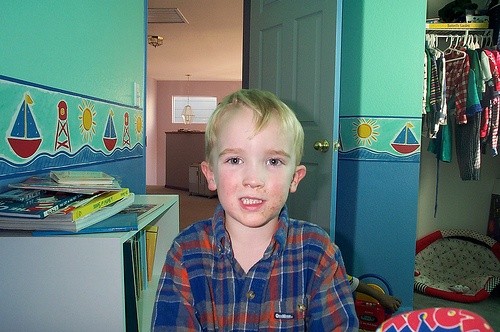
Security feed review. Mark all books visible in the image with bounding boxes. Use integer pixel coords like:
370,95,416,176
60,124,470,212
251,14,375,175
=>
123,225,158,332
0,171,163,235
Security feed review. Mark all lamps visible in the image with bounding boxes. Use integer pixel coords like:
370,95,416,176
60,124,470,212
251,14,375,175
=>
181,75,195,127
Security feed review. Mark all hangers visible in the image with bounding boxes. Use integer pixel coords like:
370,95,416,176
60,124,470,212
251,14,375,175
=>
424,24,499,67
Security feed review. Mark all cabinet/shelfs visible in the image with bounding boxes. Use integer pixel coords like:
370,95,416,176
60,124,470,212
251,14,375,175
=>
1,193,179,332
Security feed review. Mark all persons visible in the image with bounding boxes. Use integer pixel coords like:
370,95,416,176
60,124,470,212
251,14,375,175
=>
346,273,402,313
150,88,360,332
